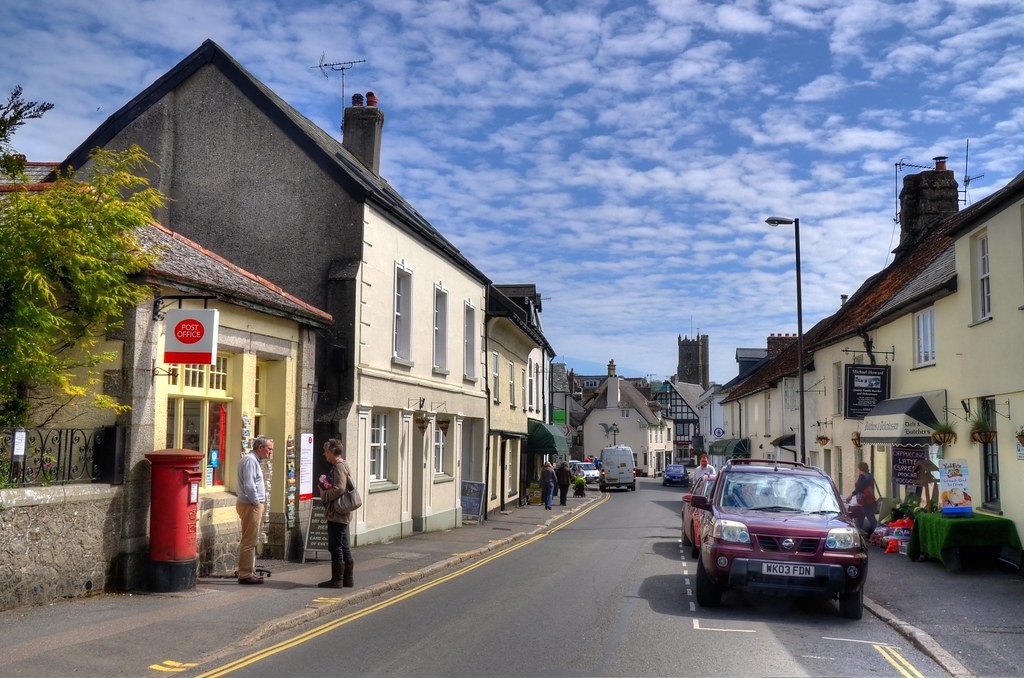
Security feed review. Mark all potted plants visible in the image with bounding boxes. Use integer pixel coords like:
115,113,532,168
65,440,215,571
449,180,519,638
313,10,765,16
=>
929,421,955,443
970,410,997,444
1015,426,1024,446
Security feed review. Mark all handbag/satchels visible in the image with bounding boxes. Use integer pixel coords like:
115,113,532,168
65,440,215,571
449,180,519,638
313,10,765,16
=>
875,498,884,513
334,479,362,513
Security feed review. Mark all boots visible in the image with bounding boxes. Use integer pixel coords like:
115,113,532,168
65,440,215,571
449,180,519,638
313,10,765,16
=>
343,560,354,588
317,562,343,589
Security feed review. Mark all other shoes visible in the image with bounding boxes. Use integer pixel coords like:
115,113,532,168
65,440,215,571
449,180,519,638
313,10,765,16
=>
239,576,263,584
545,506,551,510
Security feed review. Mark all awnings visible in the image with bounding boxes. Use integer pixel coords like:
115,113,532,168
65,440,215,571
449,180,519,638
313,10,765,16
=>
859,389,947,448
708,438,748,456
528,419,569,455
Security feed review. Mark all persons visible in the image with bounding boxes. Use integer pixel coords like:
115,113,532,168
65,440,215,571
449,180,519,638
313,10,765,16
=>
585,456,591,462
236,437,274,584
845,462,877,530
692,454,716,487
574,466,586,478
539,461,557,511
593,457,599,466
556,462,572,506
317,439,355,589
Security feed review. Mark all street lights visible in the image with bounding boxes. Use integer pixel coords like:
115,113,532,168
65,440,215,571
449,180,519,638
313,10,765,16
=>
764,214,807,468
612,422,618,446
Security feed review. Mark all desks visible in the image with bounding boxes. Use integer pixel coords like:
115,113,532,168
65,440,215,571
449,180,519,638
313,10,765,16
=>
907,513,1023,571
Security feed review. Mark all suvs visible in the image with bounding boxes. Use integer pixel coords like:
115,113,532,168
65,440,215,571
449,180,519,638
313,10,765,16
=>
689,458,869,620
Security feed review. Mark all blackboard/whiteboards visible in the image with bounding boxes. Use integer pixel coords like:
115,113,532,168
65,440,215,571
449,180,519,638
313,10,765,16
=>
891,445,927,485
304,497,330,551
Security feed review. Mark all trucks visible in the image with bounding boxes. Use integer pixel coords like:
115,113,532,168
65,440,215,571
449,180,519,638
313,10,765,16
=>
598,442,637,491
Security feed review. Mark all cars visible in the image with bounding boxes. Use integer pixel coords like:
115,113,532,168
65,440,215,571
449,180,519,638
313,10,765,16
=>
662,464,691,488
680,472,717,560
568,460,601,483
